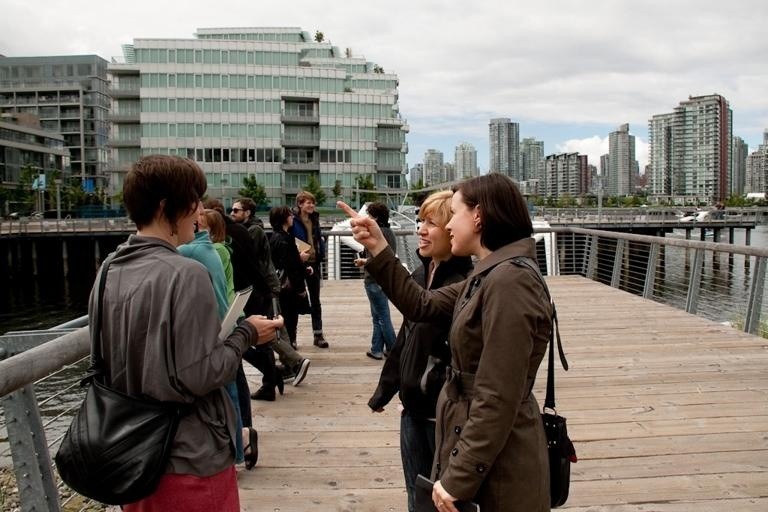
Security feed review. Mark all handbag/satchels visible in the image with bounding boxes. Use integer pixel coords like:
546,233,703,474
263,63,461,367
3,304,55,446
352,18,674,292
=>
275,269,292,292
55,379,176,505
542,413,579,508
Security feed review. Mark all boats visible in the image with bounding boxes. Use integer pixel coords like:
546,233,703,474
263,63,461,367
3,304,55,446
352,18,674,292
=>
680,209,768,222
327,200,561,282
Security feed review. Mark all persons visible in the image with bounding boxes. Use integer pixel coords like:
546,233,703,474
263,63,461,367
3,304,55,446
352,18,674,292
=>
289,191,330,349
353,201,396,361
178,198,311,473
334,173,555,512
88,156,285,512
366,189,473,512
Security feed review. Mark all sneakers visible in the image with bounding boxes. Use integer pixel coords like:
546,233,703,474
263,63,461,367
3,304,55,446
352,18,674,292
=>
366,351,389,359
313,333,328,347
250,341,310,401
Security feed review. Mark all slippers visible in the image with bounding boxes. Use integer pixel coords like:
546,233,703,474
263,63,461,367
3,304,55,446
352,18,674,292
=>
244,427,258,470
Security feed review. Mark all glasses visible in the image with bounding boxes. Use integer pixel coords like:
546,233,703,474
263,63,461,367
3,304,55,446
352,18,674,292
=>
230,208,243,213
